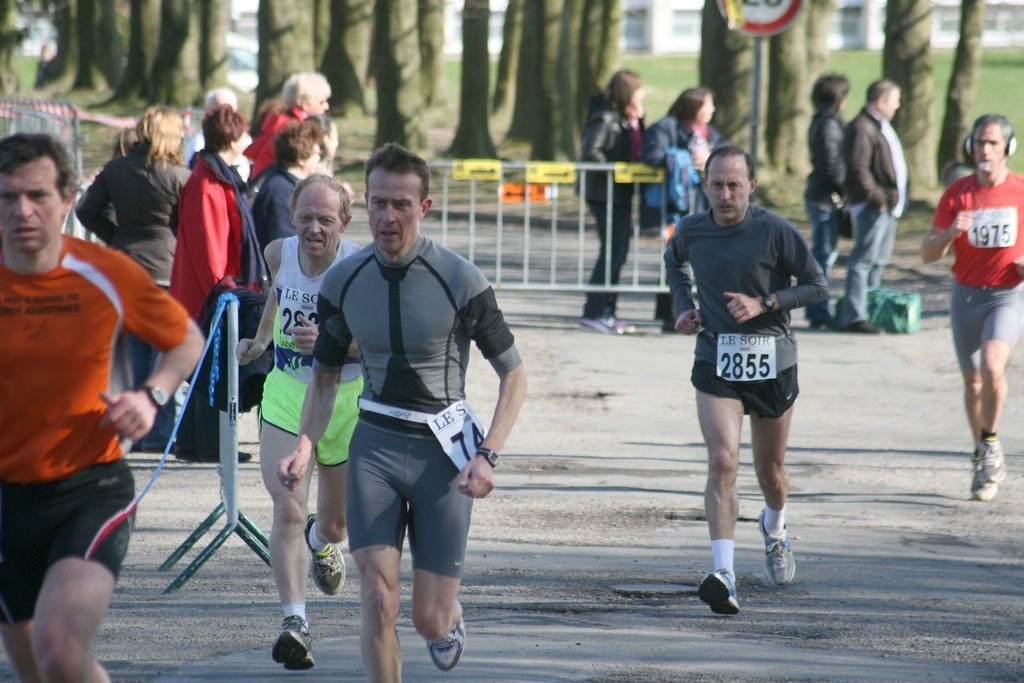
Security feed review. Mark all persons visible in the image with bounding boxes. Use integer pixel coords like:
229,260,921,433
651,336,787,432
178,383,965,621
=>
242,71,331,197
107,127,132,398
250,120,322,250
169,106,271,464
834,80,910,334
234,173,365,671
306,114,351,204
641,89,725,334
75,107,192,454
277,142,528,682
0,133,206,683
580,70,646,333
921,115,1024,499
184,87,237,164
662,146,829,614
804,76,851,328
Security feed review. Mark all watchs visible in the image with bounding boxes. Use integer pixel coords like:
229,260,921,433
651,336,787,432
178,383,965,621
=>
762,294,774,313
476,448,498,468
139,386,167,408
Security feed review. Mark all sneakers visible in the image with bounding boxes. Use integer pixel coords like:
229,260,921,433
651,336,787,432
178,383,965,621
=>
427,603,466,671
970,452,998,501
303,514,346,596
759,508,795,585
272,614,315,670
697,568,741,615
978,435,1006,483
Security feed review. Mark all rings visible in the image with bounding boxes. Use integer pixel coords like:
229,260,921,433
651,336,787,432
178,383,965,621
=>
285,481,288,484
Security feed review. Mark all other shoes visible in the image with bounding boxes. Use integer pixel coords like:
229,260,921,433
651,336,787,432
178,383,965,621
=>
663,316,677,333
847,320,882,334
811,316,833,330
130,443,174,455
581,313,637,335
175,443,251,463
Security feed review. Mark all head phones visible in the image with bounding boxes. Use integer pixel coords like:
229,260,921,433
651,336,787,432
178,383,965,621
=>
963,132,1018,157
